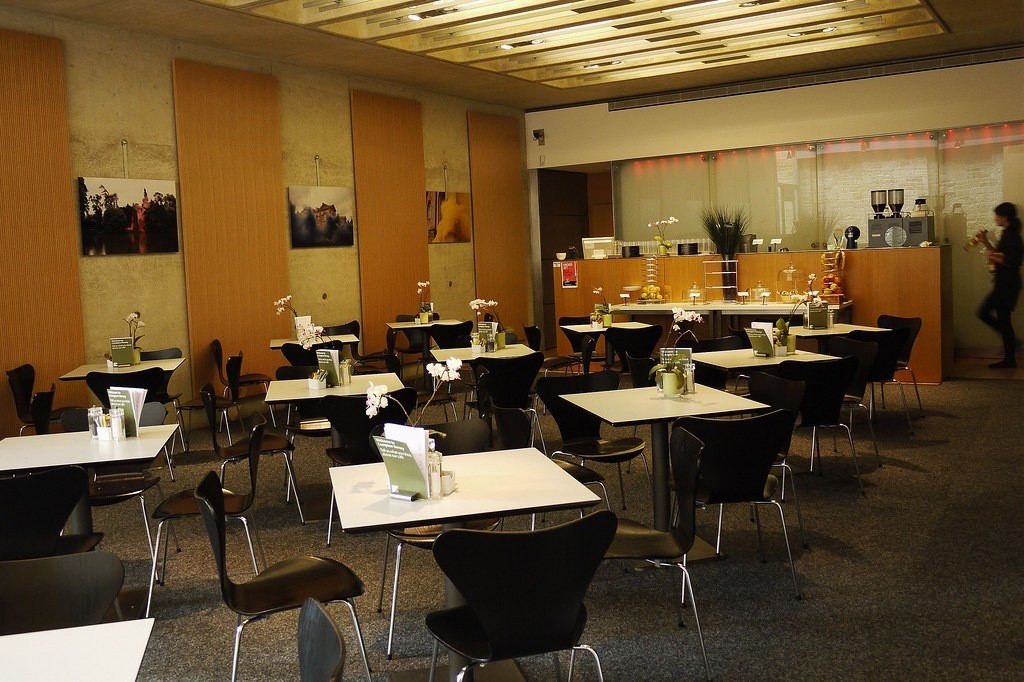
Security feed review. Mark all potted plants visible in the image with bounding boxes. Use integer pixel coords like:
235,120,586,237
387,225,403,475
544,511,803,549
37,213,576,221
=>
698,206,752,302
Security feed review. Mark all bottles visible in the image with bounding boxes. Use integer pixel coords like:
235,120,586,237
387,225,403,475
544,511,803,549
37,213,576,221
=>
487,334,494,352
88,404,102,438
109,407,126,440
342,362,351,384
590,313,597,324
428,310,433,323
428,453,442,499
829,310,834,328
682,364,695,393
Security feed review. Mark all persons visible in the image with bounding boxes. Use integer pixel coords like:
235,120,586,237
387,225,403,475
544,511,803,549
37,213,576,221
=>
974,202,1024,370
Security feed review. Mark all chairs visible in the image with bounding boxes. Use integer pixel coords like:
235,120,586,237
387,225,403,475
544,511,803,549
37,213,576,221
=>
0,312,927,682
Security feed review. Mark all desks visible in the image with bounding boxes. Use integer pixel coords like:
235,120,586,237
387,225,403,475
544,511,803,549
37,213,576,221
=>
0,617,156,682
327,447,603,682
0,424,179,537
771,323,893,356
560,321,654,367
385,319,463,391
269,334,360,350
611,300,853,336
264,372,405,468
686,346,844,373
429,344,537,364
59,358,187,381
556,381,771,570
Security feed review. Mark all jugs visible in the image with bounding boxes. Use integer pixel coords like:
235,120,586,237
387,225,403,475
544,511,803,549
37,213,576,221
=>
913,198,929,215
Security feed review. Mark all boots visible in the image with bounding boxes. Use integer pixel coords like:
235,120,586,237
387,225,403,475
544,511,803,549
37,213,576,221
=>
988,336,1018,368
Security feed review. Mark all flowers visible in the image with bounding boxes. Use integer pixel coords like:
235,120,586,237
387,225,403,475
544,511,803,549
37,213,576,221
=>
590,287,619,318
646,217,679,247
275,296,298,318
417,281,433,317
121,310,145,351
469,299,506,334
298,326,335,351
774,290,821,343
364,357,463,426
649,307,706,390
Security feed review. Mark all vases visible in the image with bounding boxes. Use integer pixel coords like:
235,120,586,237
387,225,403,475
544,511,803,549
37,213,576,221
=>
294,316,311,339
659,244,668,256
603,314,612,327
132,348,141,365
420,313,428,324
661,373,683,399
779,334,796,355
495,332,504,349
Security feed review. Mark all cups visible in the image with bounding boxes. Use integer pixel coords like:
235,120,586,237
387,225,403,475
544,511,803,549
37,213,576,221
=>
441,471,455,494
775,346,787,355
415,318,420,324
308,378,326,389
96,427,111,440
472,345,481,353
592,321,603,328
